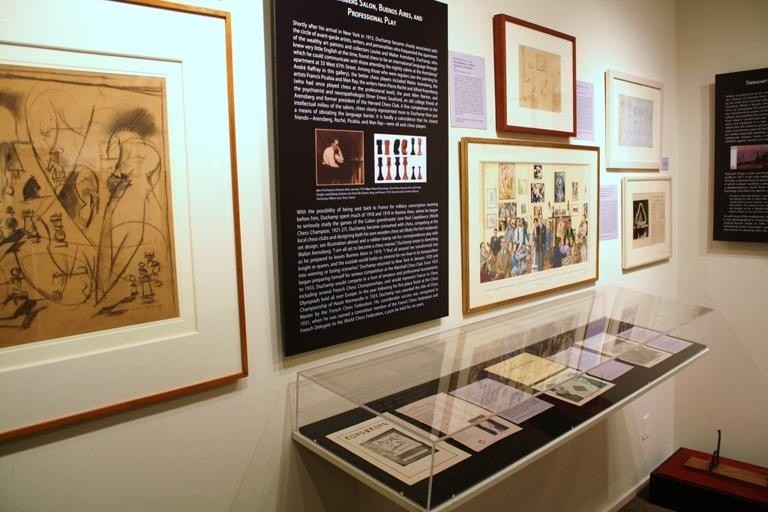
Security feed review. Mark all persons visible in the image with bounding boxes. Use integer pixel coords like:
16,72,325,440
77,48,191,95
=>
322,137,346,182
479,163,589,284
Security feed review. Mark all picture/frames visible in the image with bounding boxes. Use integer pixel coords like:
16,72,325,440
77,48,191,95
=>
620,176,673,271
492,13,577,139
605,69,664,170
461,137,601,315
0,0,249,442
